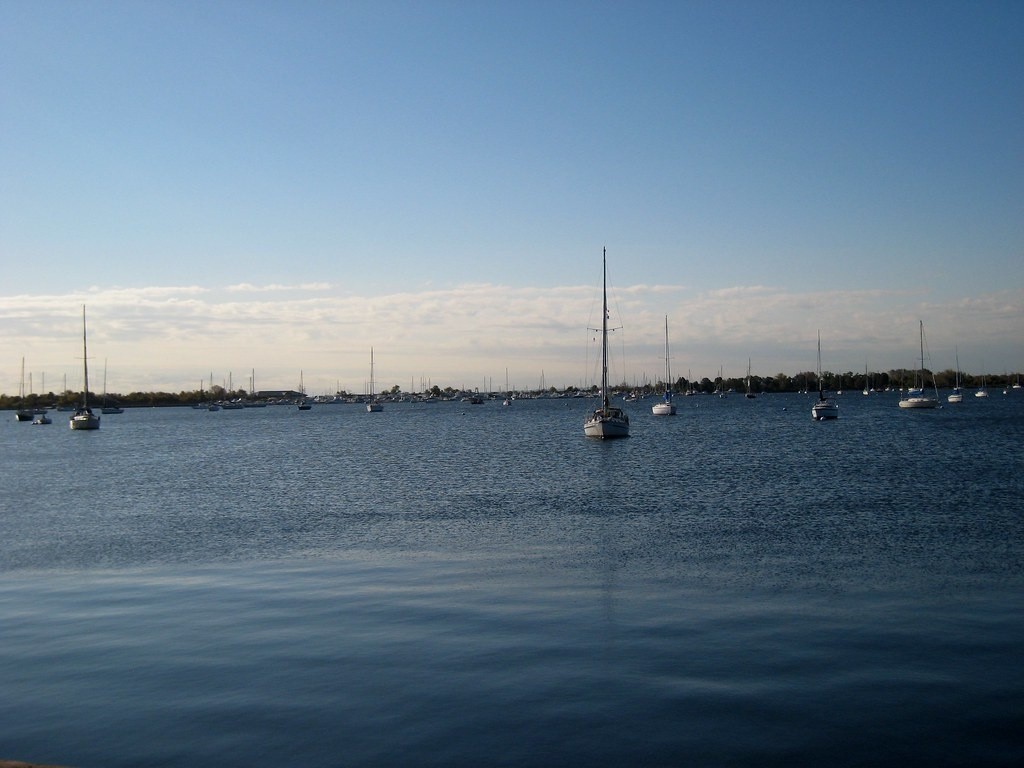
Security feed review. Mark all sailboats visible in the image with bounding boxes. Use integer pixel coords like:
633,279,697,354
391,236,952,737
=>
194,246,1024,437
16,357,73,425
70,304,101,430
102,357,124,414
